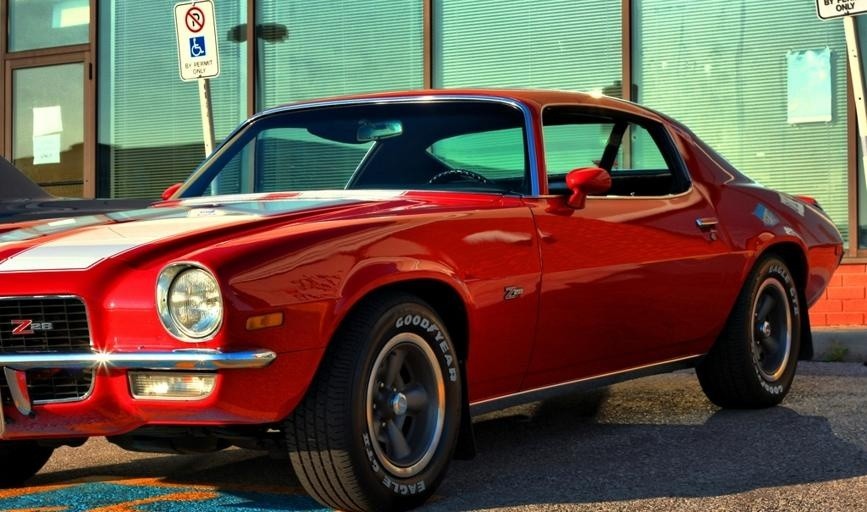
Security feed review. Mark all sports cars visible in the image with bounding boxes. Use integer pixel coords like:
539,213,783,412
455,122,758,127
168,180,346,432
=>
0,88,842,511
